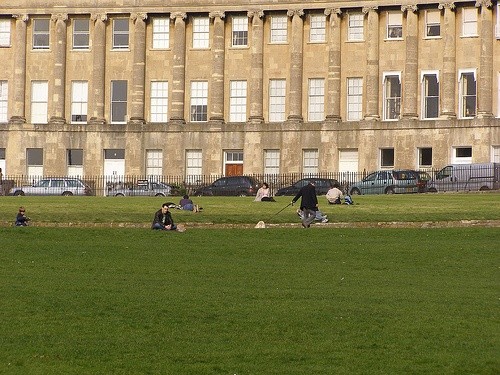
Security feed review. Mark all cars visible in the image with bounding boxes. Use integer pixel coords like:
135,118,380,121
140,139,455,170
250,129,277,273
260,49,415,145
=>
109,180,181,197
193,175,261,196
8,178,94,197
416,170,431,190
349,169,420,195
275,178,340,197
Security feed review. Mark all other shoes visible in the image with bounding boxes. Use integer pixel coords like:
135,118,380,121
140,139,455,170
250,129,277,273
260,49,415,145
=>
302,223,306,228
308,224,310,228
323,215,327,219
322,219,329,222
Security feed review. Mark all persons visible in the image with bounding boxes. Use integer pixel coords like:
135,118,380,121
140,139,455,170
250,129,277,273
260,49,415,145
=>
152,203,176,230
290,180,319,228
14,206,30,227
254,182,277,201
325,183,345,204
177,194,200,213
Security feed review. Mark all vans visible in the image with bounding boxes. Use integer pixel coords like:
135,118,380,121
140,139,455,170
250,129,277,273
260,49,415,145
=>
425,162,500,192
